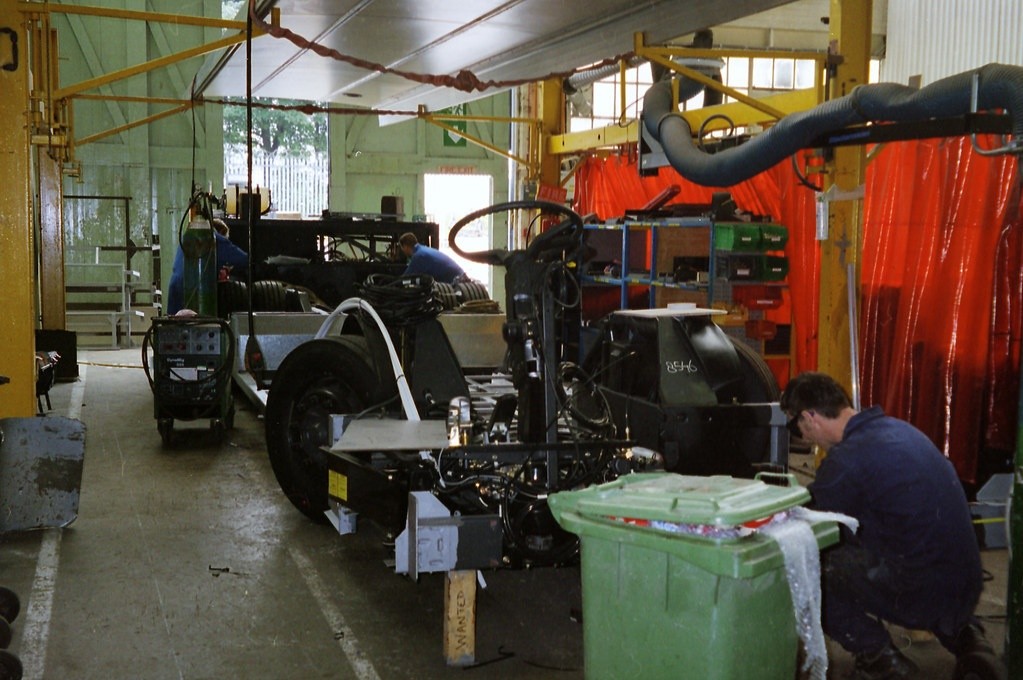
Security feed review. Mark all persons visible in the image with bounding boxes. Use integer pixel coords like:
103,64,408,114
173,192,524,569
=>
777,373,993,680
166,219,248,317
399,232,465,284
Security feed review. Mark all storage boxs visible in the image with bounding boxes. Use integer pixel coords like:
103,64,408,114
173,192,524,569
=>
673,223,790,282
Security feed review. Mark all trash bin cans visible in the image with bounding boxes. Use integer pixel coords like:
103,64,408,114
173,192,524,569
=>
548,469,841,680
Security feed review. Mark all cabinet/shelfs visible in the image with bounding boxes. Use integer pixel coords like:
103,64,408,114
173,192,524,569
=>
575,219,788,369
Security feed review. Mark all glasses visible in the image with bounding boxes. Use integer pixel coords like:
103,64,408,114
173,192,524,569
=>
786,410,814,439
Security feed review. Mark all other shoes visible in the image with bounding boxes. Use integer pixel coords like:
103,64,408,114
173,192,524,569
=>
849,641,919,680
942,619,995,656
